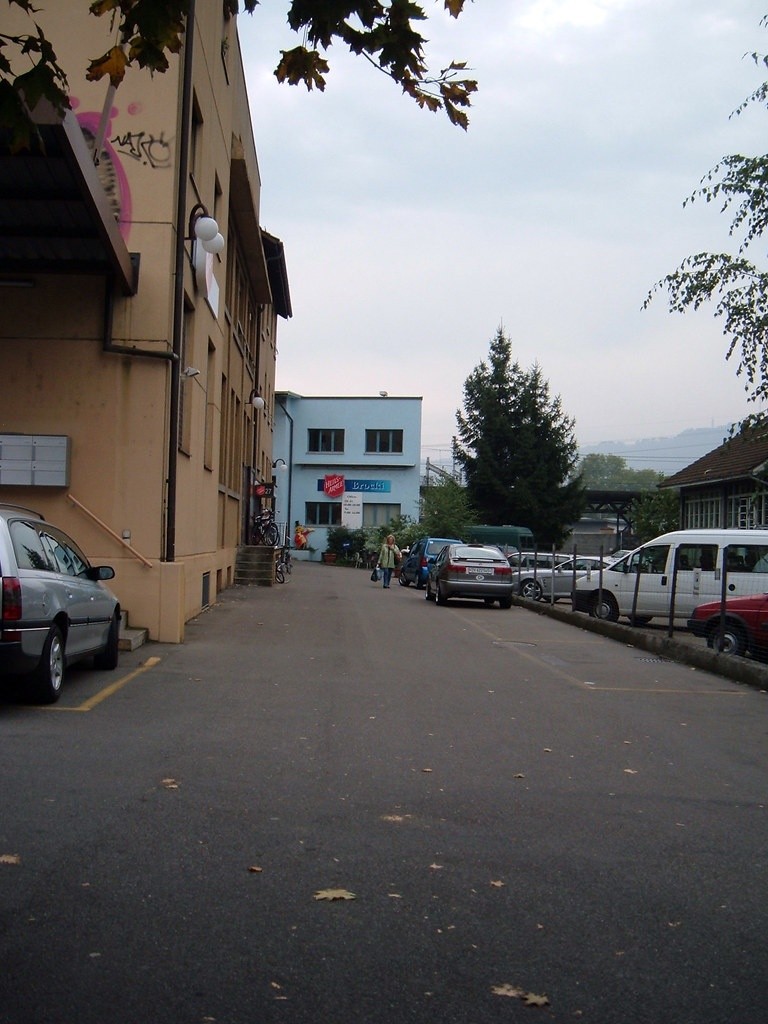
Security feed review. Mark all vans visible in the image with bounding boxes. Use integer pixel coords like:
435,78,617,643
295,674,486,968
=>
463,525,537,552
571,529,768,623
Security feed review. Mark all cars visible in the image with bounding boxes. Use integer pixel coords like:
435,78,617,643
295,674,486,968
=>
686,593,768,659
425,543,513,609
512,556,622,602
491,545,645,573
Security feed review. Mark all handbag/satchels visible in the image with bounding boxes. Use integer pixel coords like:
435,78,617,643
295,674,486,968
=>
371,564,383,582
394,544,400,565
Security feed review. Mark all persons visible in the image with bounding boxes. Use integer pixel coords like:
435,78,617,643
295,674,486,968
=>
376,535,401,589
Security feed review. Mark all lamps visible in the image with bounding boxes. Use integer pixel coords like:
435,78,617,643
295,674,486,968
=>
183,366,200,377
245,389,264,409
272,459,287,471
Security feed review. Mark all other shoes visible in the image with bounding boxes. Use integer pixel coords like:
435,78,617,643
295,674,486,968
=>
384,586,387,588
388,587,390,588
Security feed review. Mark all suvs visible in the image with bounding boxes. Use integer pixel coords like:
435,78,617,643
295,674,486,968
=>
0,501,122,706
399,537,463,589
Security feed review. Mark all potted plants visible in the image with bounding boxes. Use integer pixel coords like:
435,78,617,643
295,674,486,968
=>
395,558,405,577
320,527,337,563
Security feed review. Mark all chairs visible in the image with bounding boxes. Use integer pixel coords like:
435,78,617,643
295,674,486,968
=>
680,554,689,570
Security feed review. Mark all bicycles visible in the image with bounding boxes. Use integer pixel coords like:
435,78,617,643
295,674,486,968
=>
250,507,293,584
